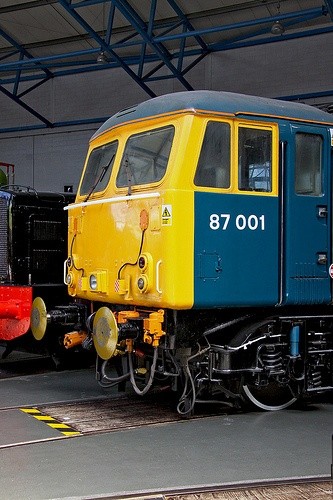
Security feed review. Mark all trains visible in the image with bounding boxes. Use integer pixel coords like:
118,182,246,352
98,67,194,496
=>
30,90,333,418
